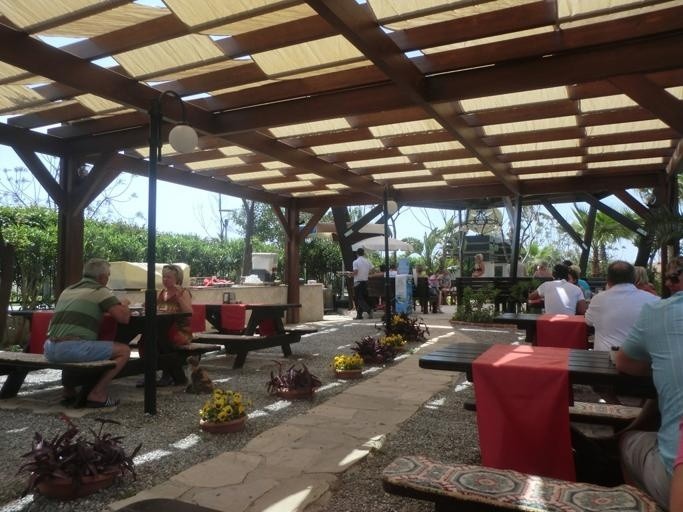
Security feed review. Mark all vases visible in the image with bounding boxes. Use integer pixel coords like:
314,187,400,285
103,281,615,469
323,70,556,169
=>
199,415,247,438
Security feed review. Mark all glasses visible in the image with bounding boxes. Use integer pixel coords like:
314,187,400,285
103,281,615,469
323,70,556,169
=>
664,271,680,283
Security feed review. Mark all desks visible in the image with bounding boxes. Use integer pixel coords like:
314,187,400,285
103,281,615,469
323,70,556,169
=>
107,281,324,333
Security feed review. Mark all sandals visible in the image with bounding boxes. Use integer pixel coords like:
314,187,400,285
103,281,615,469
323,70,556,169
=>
158,375,174,386
136,379,144,386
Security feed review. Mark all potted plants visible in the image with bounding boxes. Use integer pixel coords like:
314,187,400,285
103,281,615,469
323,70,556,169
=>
12,410,145,504
447,285,516,333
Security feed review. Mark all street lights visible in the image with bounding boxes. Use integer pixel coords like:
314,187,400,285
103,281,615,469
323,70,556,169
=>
383,184,400,340
142,86,205,417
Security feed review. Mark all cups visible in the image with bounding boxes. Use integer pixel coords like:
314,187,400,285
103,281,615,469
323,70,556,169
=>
610,350,619,365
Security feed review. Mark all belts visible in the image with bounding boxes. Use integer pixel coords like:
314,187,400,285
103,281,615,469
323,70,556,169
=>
48,334,80,342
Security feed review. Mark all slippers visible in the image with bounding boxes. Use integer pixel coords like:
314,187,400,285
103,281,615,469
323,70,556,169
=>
85,394,121,408
60,390,80,406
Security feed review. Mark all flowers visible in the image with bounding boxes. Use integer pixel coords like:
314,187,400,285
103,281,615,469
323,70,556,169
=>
198,307,431,424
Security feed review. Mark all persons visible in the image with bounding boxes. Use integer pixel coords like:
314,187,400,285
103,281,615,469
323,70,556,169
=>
472,254,484,307
349,248,456,319
527,260,591,316
633,266,659,296
43,258,131,408
507,256,552,278
614,256,683,510
583,259,663,409
136,265,193,388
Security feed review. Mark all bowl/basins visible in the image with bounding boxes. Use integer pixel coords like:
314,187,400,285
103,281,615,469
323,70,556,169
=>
128,306,144,314
211,283,234,288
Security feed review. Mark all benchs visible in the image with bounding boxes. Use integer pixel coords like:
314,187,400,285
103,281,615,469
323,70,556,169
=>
0,301,321,409
376,453,661,511
460,394,643,431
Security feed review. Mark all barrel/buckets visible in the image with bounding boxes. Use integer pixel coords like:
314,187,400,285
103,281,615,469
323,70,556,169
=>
397,258,409,274
